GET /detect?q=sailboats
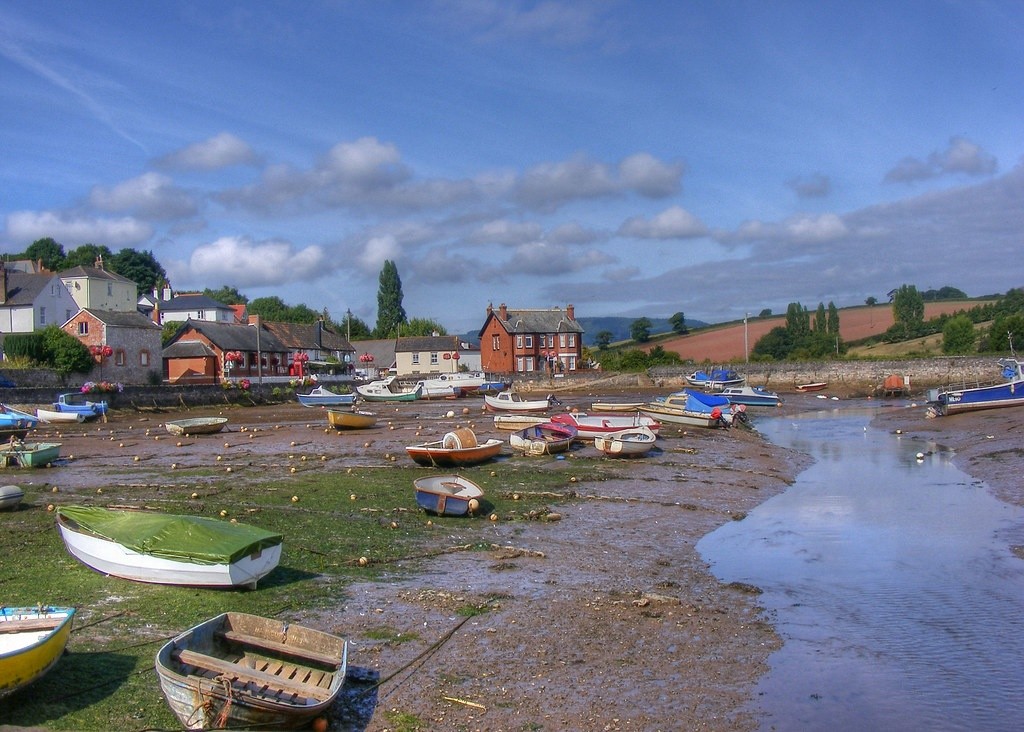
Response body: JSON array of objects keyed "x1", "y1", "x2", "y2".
[{"x1": 713, "y1": 310, "x2": 781, "y2": 405}]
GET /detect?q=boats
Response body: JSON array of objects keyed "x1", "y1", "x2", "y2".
[
  {"x1": 0, "y1": 484, "x2": 24, "y2": 510},
  {"x1": 482, "y1": 392, "x2": 561, "y2": 413},
  {"x1": 0, "y1": 404, "x2": 39, "y2": 441},
  {"x1": 406, "y1": 426, "x2": 504, "y2": 467},
  {"x1": 53, "y1": 392, "x2": 108, "y2": 418},
  {"x1": 592, "y1": 402, "x2": 647, "y2": 411},
  {"x1": 414, "y1": 473, "x2": 485, "y2": 517},
  {"x1": 0, "y1": 442, "x2": 62, "y2": 468},
  {"x1": 356, "y1": 371, "x2": 511, "y2": 401},
  {"x1": 935, "y1": 358, "x2": 1024, "y2": 416},
  {"x1": 154, "y1": 611, "x2": 347, "y2": 732},
  {"x1": 795, "y1": 381, "x2": 827, "y2": 393},
  {"x1": 295, "y1": 386, "x2": 356, "y2": 407},
  {"x1": 327, "y1": 406, "x2": 376, "y2": 429},
  {"x1": 0, "y1": 607, "x2": 75, "y2": 698},
  {"x1": 884, "y1": 374, "x2": 905, "y2": 389},
  {"x1": 636, "y1": 368, "x2": 747, "y2": 427},
  {"x1": 494, "y1": 411, "x2": 662, "y2": 455},
  {"x1": 35, "y1": 407, "x2": 85, "y2": 422},
  {"x1": 55, "y1": 505, "x2": 283, "y2": 589},
  {"x1": 165, "y1": 416, "x2": 228, "y2": 435}
]
